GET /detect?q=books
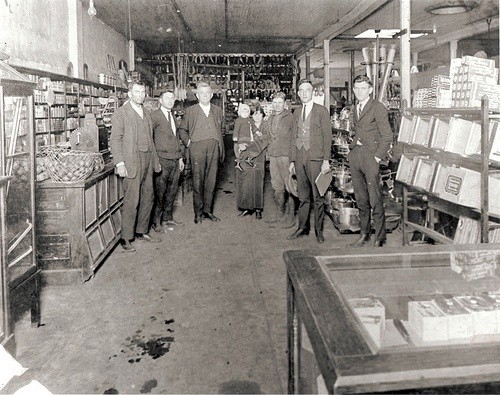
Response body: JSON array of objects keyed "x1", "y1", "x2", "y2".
[{"x1": 315, "y1": 166, "x2": 332, "y2": 196}]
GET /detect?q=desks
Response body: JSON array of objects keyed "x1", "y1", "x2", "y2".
[{"x1": 282, "y1": 243, "x2": 500, "y2": 395}]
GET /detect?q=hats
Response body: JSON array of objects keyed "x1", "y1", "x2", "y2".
[
  {"x1": 285, "y1": 169, "x2": 298, "y2": 198},
  {"x1": 237, "y1": 104, "x2": 251, "y2": 116}
]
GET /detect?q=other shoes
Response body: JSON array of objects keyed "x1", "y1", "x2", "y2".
[
  {"x1": 357, "y1": 232, "x2": 370, "y2": 243},
  {"x1": 234, "y1": 162, "x2": 244, "y2": 171},
  {"x1": 287, "y1": 226, "x2": 310, "y2": 239},
  {"x1": 194, "y1": 214, "x2": 202, "y2": 224},
  {"x1": 256, "y1": 209, "x2": 262, "y2": 219},
  {"x1": 374, "y1": 240, "x2": 384, "y2": 247},
  {"x1": 245, "y1": 157, "x2": 254, "y2": 167},
  {"x1": 317, "y1": 235, "x2": 324, "y2": 242},
  {"x1": 120, "y1": 238, "x2": 136, "y2": 252},
  {"x1": 152, "y1": 221, "x2": 164, "y2": 233},
  {"x1": 137, "y1": 232, "x2": 161, "y2": 243},
  {"x1": 162, "y1": 219, "x2": 185, "y2": 226},
  {"x1": 204, "y1": 212, "x2": 221, "y2": 222},
  {"x1": 239, "y1": 209, "x2": 254, "y2": 217}
]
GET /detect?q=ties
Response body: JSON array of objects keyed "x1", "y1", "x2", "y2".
[
  {"x1": 167, "y1": 110, "x2": 171, "y2": 127},
  {"x1": 358, "y1": 104, "x2": 363, "y2": 118},
  {"x1": 303, "y1": 104, "x2": 306, "y2": 121}
]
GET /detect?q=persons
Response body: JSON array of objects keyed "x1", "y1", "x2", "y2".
[
  {"x1": 233, "y1": 104, "x2": 262, "y2": 171},
  {"x1": 110, "y1": 81, "x2": 163, "y2": 251},
  {"x1": 288, "y1": 80, "x2": 333, "y2": 243},
  {"x1": 266, "y1": 91, "x2": 299, "y2": 228},
  {"x1": 347, "y1": 75, "x2": 393, "y2": 247},
  {"x1": 148, "y1": 90, "x2": 184, "y2": 233},
  {"x1": 178, "y1": 82, "x2": 227, "y2": 225},
  {"x1": 234, "y1": 104, "x2": 269, "y2": 219}
]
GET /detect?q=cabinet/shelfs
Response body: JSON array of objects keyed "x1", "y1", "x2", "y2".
[
  {"x1": 394, "y1": 96, "x2": 500, "y2": 249},
  {"x1": 0, "y1": 56, "x2": 132, "y2": 340}
]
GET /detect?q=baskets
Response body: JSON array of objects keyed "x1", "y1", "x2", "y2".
[{"x1": 43, "y1": 148, "x2": 106, "y2": 182}]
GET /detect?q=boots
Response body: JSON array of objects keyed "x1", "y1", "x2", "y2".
[
  {"x1": 282, "y1": 198, "x2": 296, "y2": 228},
  {"x1": 266, "y1": 190, "x2": 285, "y2": 222}
]
